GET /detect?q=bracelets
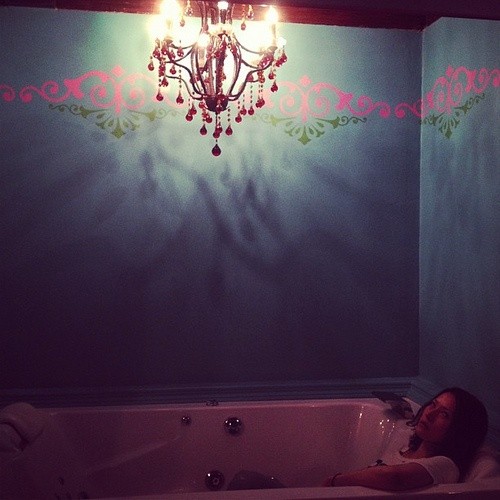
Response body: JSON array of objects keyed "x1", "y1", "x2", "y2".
[{"x1": 331, "y1": 472, "x2": 343, "y2": 486}]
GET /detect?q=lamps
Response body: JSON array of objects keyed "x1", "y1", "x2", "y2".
[{"x1": 147, "y1": 0, "x2": 287, "y2": 156}]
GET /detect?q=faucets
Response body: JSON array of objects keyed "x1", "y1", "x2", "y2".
[{"x1": 373, "y1": 389, "x2": 413, "y2": 422}]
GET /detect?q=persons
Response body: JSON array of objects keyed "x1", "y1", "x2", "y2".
[{"x1": 222, "y1": 386, "x2": 488, "y2": 491}]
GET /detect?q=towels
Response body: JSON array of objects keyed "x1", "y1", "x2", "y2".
[{"x1": 0, "y1": 402, "x2": 48, "y2": 452}]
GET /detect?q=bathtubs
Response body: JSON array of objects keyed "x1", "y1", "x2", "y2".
[{"x1": 0, "y1": 397, "x2": 500, "y2": 500}]
